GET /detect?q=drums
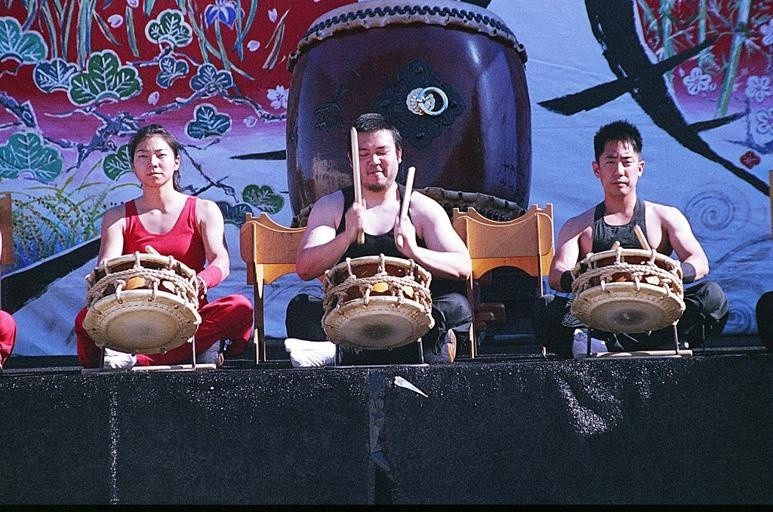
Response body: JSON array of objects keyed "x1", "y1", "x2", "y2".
[
  {"x1": 284, "y1": 0, "x2": 531, "y2": 227},
  {"x1": 320, "y1": 253, "x2": 435, "y2": 355},
  {"x1": 80, "y1": 250, "x2": 201, "y2": 357},
  {"x1": 570, "y1": 247, "x2": 686, "y2": 336}
]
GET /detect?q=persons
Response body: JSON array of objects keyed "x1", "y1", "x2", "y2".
[
  {"x1": 68, "y1": 122, "x2": 254, "y2": 371},
  {"x1": 530, "y1": 121, "x2": 730, "y2": 357},
  {"x1": 280, "y1": 110, "x2": 476, "y2": 366}
]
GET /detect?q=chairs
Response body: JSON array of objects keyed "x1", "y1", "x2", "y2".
[
  {"x1": 238, "y1": 212, "x2": 325, "y2": 366},
  {"x1": 451, "y1": 201, "x2": 557, "y2": 360}
]
